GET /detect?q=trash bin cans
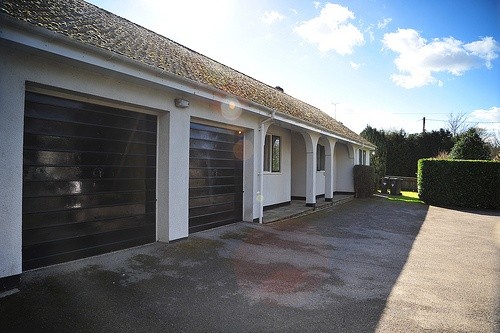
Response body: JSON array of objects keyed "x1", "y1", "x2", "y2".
[
  {"x1": 389, "y1": 178, "x2": 404, "y2": 196},
  {"x1": 381, "y1": 177, "x2": 390, "y2": 194}
]
